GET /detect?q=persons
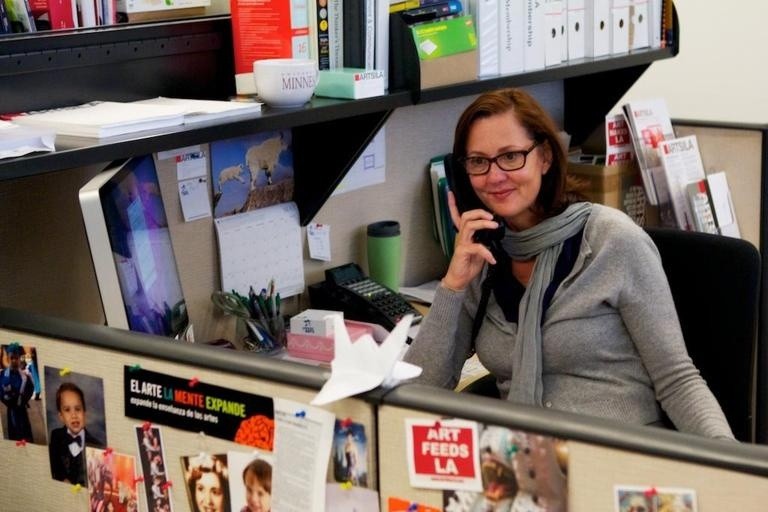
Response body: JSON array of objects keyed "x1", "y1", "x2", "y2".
[
  {"x1": 0, "y1": 345, "x2": 35, "y2": 444},
  {"x1": 344, "y1": 431, "x2": 362, "y2": 487},
  {"x1": 184, "y1": 452, "x2": 231, "y2": 511},
  {"x1": 49, "y1": 382, "x2": 102, "y2": 487},
  {"x1": 239, "y1": 458, "x2": 272, "y2": 512},
  {"x1": 399, "y1": 86, "x2": 738, "y2": 442},
  {"x1": 140, "y1": 429, "x2": 170, "y2": 511},
  {"x1": 89, "y1": 476, "x2": 136, "y2": 512}
]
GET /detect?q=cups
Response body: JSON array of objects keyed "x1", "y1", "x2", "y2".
[{"x1": 251, "y1": 57, "x2": 323, "y2": 110}]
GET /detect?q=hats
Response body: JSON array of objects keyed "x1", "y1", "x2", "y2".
[{"x1": 4, "y1": 346, "x2": 26, "y2": 356}]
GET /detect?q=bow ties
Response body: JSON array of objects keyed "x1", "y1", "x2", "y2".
[{"x1": 66, "y1": 432, "x2": 83, "y2": 448}]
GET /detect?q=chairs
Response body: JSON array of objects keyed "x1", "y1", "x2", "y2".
[{"x1": 459, "y1": 228, "x2": 760, "y2": 443}]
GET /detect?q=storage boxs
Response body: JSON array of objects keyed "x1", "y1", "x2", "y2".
[{"x1": 566, "y1": 160, "x2": 659, "y2": 228}]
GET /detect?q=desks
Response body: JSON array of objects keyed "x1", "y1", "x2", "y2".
[{"x1": 0, "y1": 272, "x2": 768, "y2": 512}]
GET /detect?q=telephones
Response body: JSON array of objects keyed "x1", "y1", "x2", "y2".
[{"x1": 443, "y1": 152, "x2": 506, "y2": 242}]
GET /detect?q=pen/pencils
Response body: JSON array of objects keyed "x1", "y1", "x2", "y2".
[{"x1": 232, "y1": 279, "x2": 281, "y2": 349}]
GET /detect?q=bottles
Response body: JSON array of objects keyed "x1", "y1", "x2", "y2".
[{"x1": 366, "y1": 218, "x2": 401, "y2": 299}]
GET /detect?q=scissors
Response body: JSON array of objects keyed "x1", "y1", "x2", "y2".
[{"x1": 211, "y1": 292, "x2": 251, "y2": 319}]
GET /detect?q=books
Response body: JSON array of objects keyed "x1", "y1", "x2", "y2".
[
  {"x1": 0, "y1": 1, "x2": 211, "y2": 36},
  {"x1": 618, "y1": 96, "x2": 742, "y2": 239},
  {"x1": 227, "y1": 1, "x2": 392, "y2": 97},
  {"x1": 0, "y1": 95, "x2": 266, "y2": 153}
]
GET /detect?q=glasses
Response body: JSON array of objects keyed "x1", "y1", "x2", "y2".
[{"x1": 457, "y1": 136, "x2": 544, "y2": 177}]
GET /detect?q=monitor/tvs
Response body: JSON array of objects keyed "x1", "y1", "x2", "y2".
[{"x1": 76, "y1": 152, "x2": 192, "y2": 341}]
